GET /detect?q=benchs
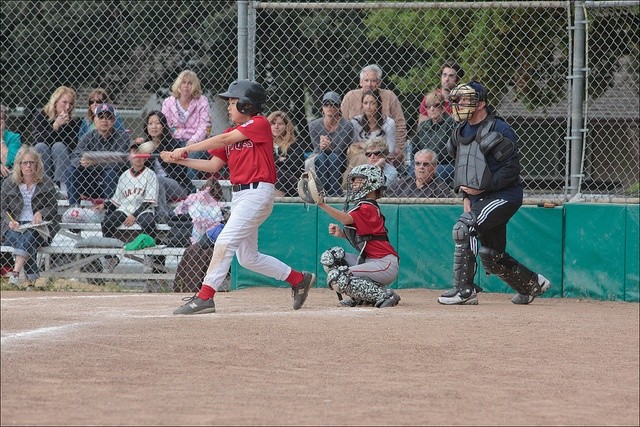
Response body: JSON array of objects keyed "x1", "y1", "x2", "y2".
[
  {"x1": 1, "y1": 245, "x2": 186, "y2": 280},
  {"x1": 58, "y1": 222, "x2": 171, "y2": 232},
  {"x1": 56, "y1": 200, "x2": 93, "y2": 217},
  {"x1": 191, "y1": 178, "x2": 234, "y2": 204}
]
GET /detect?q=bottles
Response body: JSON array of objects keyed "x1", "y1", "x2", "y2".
[{"x1": 404, "y1": 139, "x2": 413, "y2": 160}]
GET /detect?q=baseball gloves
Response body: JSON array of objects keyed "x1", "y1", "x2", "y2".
[{"x1": 297, "y1": 170, "x2": 325, "y2": 204}]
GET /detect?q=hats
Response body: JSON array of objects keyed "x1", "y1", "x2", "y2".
[
  {"x1": 322, "y1": 91, "x2": 342, "y2": 104},
  {"x1": 126, "y1": 138, "x2": 145, "y2": 152},
  {"x1": 96, "y1": 103, "x2": 114, "y2": 117}
]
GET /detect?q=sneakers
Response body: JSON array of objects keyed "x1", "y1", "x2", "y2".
[
  {"x1": 291, "y1": 271, "x2": 315, "y2": 310},
  {"x1": 173, "y1": 291, "x2": 215, "y2": 314},
  {"x1": 337, "y1": 297, "x2": 357, "y2": 306},
  {"x1": 143, "y1": 257, "x2": 155, "y2": 273},
  {"x1": 7, "y1": 275, "x2": 20, "y2": 289},
  {"x1": 103, "y1": 256, "x2": 120, "y2": 273},
  {"x1": 437, "y1": 287, "x2": 479, "y2": 305},
  {"x1": 511, "y1": 273, "x2": 552, "y2": 304},
  {"x1": 17, "y1": 274, "x2": 29, "y2": 289},
  {"x1": 379, "y1": 289, "x2": 401, "y2": 308}
]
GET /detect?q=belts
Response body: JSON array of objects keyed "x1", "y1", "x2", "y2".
[{"x1": 230, "y1": 182, "x2": 259, "y2": 191}]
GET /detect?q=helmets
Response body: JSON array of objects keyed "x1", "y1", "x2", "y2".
[
  {"x1": 345, "y1": 164, "x2": 386, "y2": 202},
  {"x1": 218, "y1": 79, "x2": 267, "y2": 114},
  {"x1": 448, "y1": 81, "x2": 488, "y2": 124}
]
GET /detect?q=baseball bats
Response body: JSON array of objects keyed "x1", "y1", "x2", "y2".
[{"x1": 82, "y1": 150, "x2": 189, "y2": 162}]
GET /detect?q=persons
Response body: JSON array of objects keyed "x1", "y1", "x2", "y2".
[
  {"x1": 160, "y1": 80, "x2": 316, "y2": 315},
  {"x1": 342, "y1": 65, "x2": 408, "y2": 167},
  {"x1": 341, "y1": 89, "x2": 399, "y2": 192},
  {"x1": 383, "y1": 150, "x2": 459, "y2": 199},
  {"x1": 65, "y1": 104, "x2": 130, "y2": 232},
  {"x1": 268, "y1": 111, "x2": 305, "y2": 196},
  {"x1": 32, "y1": 85, "x2": 82, "y2": 198},
  {"x1": 363, "y1": 139, "x2": 400, "y2": 198},
  {"x1": 298, "y1": 164, "x2": 401, "y2": 308},
  {"x1": 406, "y1": 92, "x2": 461, "y2": 187},
  {"x1": 1, "y1": 146, "x2": 58, "y2": 290},
  {"x1": 308, "y1": 92, "x2": 354, "y2": 197},
  {"x1": 140, "y1": 110, "x2": 196, "y2": 248},
  {"x1": 437, "y1": 80, "x2": 550, "y2": 304},
  {"x1": 77, "y1": 88, "x2": 125, "y2": 145},
  {"x1": 102, "y1": 140, "x2": 166, "y2": 243},
  {"x1": 418, "y1": 62, "x2": 465, "y2": 127},
  {"x1": 161, "y1": 70, "x2": 212, "y2": 179},
  {"x1": 0, "y1": 111, "x2": 21, "y2": 276}
]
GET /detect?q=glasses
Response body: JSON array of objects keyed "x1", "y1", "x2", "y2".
[
  {"x1": 21, "y1": 161, "x2": 36, "y2": 165},
  {"x1": 425, "y1": 103, "x2": 441, "y2": 108},
  {"x1": 365, "y1": 151, "x2": 385, "y2": 157},
  {"x1": 415, "y1": 162, "x2": 433, "y2": 167},
  {"x1": 90, "y1": 99, "x2": 102, "y2": 104},
  {"x1": 96, "y1": 113, "x2": 112, "y2": 119},
  {"x1": 323, "y1": 101, "x2": 338, "y2": 107}
]
